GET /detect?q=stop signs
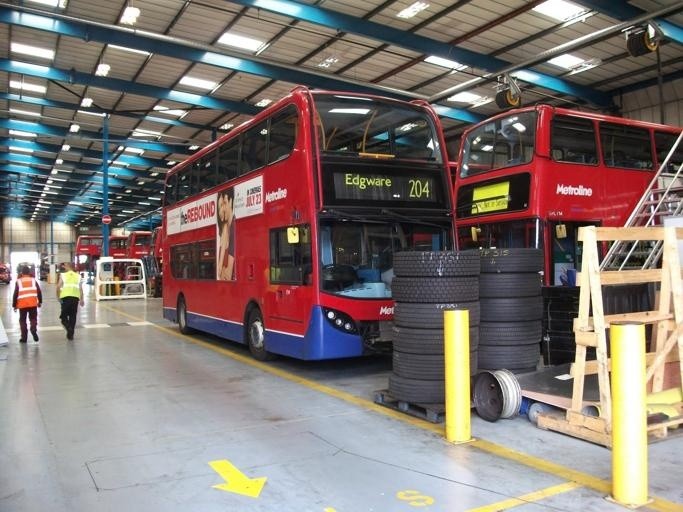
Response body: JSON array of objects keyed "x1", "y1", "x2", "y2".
[{"x1": 102, "y1": 216, "x2": 112, "y2": 224}]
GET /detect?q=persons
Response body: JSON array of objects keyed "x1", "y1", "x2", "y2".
[
  {"x1": 55, "y1": 262, "x2": 85, "y2": 341},
  {"x1": 217, "y1": 191, "x2": 230, "y2": 281},
  {"x1": 223, "y1": 187, "x2": 236, "y2": 281},
  {"x1": 12, "y1": 267, "x2": 43, "y2": 344}
]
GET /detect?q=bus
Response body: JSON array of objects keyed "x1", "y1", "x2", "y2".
[
  {"x1": 72, "y1": 225, "x2": 164, "y2": 296},
  {"x1": 453, "y1": 105, "x2": 683, "y2": 287},
  {"x1": 158, "y1": 90, "x2": 454, "y2": 360}
]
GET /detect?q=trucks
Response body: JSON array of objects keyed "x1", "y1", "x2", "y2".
[
  {"x1": 17, "y1": 261, "x2": 71, "y2": 281},
  {"x1": 0, "y1": 262, "x2": 11, "y2": 284}
]
